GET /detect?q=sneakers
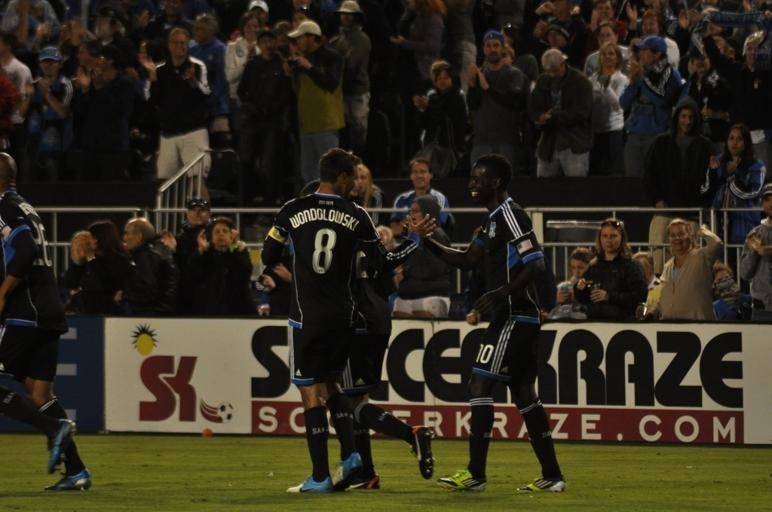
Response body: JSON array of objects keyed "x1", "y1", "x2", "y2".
[
  {"x1": 286, "y1": 474, "x2": 334, "y2": 494},
  {"x1": 515, "y1": 475, "x2": 567, "y2": 493},
  {"x1": 48, "y1": 418, "x2": 76, "y2": 474},
  {"x1": 436, "y1": 469, "x2": 487, "y2": 492},
  {"x1": 332, "y1": 451, "x2": 379, "y2": 492},
  {"x1": 407, "y1": 425, "x2": 434, "y2": 479},
  {"x1": 44, "y1": 467, "x2": 92, "y2": 491}
]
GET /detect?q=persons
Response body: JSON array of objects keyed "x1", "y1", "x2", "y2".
[
  {"x1": 57, "y1": 230, "x2": 97, "y2": 311},
  {"x1": 389, "y1": 157, "x2": 451, "y2": 245},
  {"x1": 672, "y1": 0, "x2": 772, "y2": 141},
  {"x1": 401, "y1": 154, "x2": 568, "y2": 492},
  {"x1": 300, "y1": 179, "x2": 434, "y2": 489},
  {"x1": 189, "y1": 216, "x2": 255, "y2": 315},
  {"x1": 632, "y1": 251, "x2": 662, "y2": 300},
  {"x1": 348, "y1": 164, "x2": 391, "y2": 228},
  {"x1": 547, "y1": 247, "x2": 595, "y2": 321},
  {"x1": 381, "y1": 194, "x2": 451, "y2": 318},
  {"x1": 172, "y1": 198, "x2": 216, "y2": 318},
  {"x1": 572, "y1": 218, "x2": 648, "y2": 323},
  {"x1": 1, "y1": 1, "x2": 371, "y2": 207},
  {"x1": 644, "y1": 95, "x2": 717, "y2": 279},
  {"x1": 369, "y1": 1, "x2": 672, "y2": 175},
  {"x1": 89, "y1": 218, "x2": 127, "y2": 317},
  {"x1": 113, "y1": 216, "x2": 178, "y2": 317},
  {"x1": 0, "y1": 152, "x2": 92, "y2": 492},
  {"x1": 657, "y1": 216, "x2": 724, "y2": 322},
  {"x1": 739, "y1": 182, "x2": 772, "y2": 325},
  {"x1": 712, "y1": 260, "x2": 752, "y2": 323},
  {"x1": 698, "y1": 123, "x2": 766, "y2": 292},
  {"x1": 260, "y1": 148, "x2": 436, "y2": 493}
]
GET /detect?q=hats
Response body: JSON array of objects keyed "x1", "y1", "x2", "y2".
[
  {"x1": 757, "y1": 184, "x2": 772, "y2": 199},
  {"x1": 635, "y1": 36, "x2": 667, "y2": 52},
  {"x1": 540, "y1": 49, "x2": 569, "y2": 71},
  {"x1": 249, "y1": 1, "x2": 270, "y2": 14},
  {"x1": 287, "y1": 21, "x2": 322, "y2": 39},
  {"x1": 185, "y1": 199, "x2": 211, "y2": 211},
  {"x1": 483, "y1": 31, "x2": 505, "y2": 45},
  {"x1": 335, "y1": 1, "x2": 363, "y2": 14},
  {"x1": 38, "y1": 47, "x2": 64, "y2": 63}
]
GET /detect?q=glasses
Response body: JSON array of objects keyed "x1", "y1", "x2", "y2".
[
  {"x1": 189, "y1": 201, "x2": 205, "y2": 206},
  {"x1": 601, "y1": 221, "x2": 622, "y2": 228}
]
